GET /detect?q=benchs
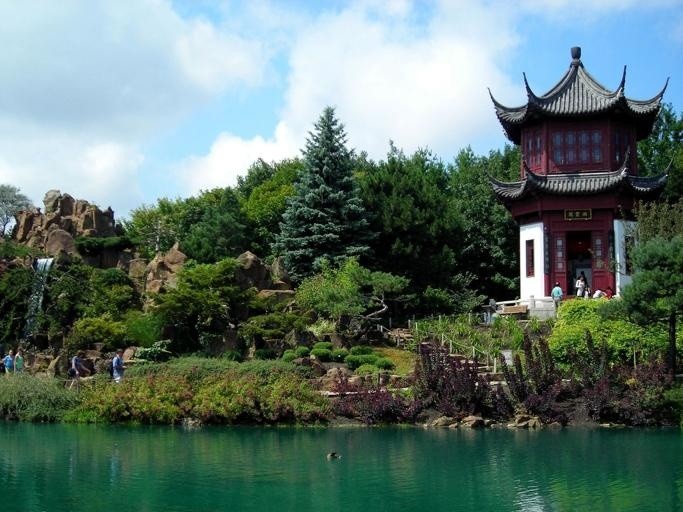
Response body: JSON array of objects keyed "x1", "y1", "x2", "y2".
[{"x1": 499, "y1": 305, "x2": 528, "y2": 320}]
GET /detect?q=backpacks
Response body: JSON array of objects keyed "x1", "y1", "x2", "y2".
[{"x1": 110, "y1": 357, "x2": 119, "y2": 375}]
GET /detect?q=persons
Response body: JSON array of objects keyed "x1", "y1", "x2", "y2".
[
  {"x1": 550, "y1": 282, "x2": 563, "y2": 312},
  {"x1": 112, "y1": 348, "x2": 128, "y2": 384},
  {"x1": 0, "y1": 350, "x2": 15, "y2": 375},
  {"x1": 576, "y1": 272, "x2": 614, "y2": 300},
  {"x1": 68, "y1": 351, "x2": 91, "y2": 395},
  {"x1": 15, "y1": 348, "x2": 25, "y2": 373}
]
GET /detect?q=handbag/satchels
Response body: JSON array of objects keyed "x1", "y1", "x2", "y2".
[{"x1": 67, "y1": 368, "x2": 76, "y2": 377}]
